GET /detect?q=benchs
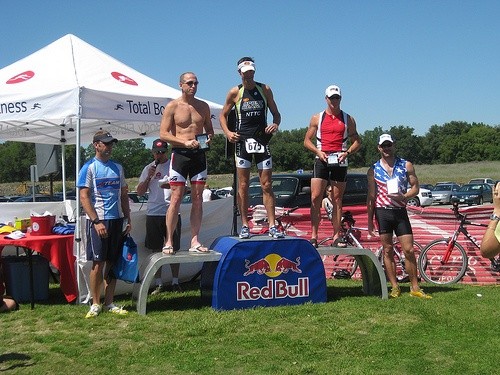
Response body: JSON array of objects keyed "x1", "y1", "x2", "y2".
[{"x1": 129, "y1": 234, "x2": 389, "y2": 314}]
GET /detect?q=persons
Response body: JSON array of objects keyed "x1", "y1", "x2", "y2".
[
  {"x1": 302, "y1": 85, "x2": 364, "y2": 248},
  {"x1": 158, "y1": 71, "x2": 212, "y2": 256},
  {"x1": 479, "y1": 180, "x2": 500, "y2": 259},
  {"x1": 365, "y1": 133, "x2": 435, "y2": 302},
  {"x1": 137, "y1": 137, "x2": 184, "y2": 297},
  {"x1": 74, "y1": 130, "x2": 133, "y2": 319},
  {"x1": 321, "y1": 184, "x2": 334, "y2": 210},
  {"x1": 220, "y1": 56, "x2": 286, "y2": 240}
]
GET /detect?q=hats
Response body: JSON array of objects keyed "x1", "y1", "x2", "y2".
[
  {"x1": 376, "y1": 134, "x2": 394, "y2": 147},
  {"x1": 151, "y1": 139, "x2": 168, "y2": 153},
  {"x1": 237, "y1": 61, "x2": 256, "y2": 73},
  {"x1": 92, "y1": 132, "x2": 118, "y2": 144},
  {"x1": 324, "y1": 85, "x2": 341, "y2": 98}
]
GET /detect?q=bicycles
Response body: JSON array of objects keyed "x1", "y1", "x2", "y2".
[
  {"x1": 313, "y1": 211, "x2": 426, "y2": 282},
  {"x1": 417, "y1": 198, "x2": 500, "y2": 287}
]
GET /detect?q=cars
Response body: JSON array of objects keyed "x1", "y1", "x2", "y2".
[{"x1": 232, "y1": 167, "x2": 498, "y2": 216}]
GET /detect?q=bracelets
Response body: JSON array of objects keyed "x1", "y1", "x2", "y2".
[
  {"x1": 346, "y1": 150, "x2": 351, "y2": 155},
  {"x1": 489, "y1": 212, "x2": 500, "y2": 221},
  {"x1": 92, "y1": 218, "x2": 100, "y2": 224}
]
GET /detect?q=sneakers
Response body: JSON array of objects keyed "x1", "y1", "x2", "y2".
[
  {"x1": 268, "y1": 226, "x2": 285, "y2": 239},
  {"x1": 102, "y1": 302, "x2": 129, "y2": 316},
  {"x1": 409, "y1": 286, "x2": 433, "y2": 301},
  {"x1": 85, "y1": 304, "x2": 101, "y2": 318},
  {"x1": 389, "y1": 286, "x2": 401, "y2": 298},
  {"x1": 239, "y1": 225, "x2": 251, "y2": 239}
]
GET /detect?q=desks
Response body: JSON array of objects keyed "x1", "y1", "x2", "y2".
[{"x1": 0, "y1": 224, "x2": 75, "y2": 309}]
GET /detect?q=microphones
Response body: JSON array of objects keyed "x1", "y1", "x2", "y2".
[{"x1": 149, "y1": 160, "x2": 159, "y2": 179}]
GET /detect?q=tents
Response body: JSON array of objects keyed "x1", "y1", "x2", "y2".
[{"x1": 0, "y1": 32, "x2": 233, "y2": 302}]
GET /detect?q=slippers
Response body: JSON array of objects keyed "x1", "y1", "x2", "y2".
[
  {"x1": 309, "y1": 239, "x2": 318, "y2": 248},
  {"x1": 162, "y1": 246, "x2": 176, "y2": 255},
  {"x1": 188, "y1": 244, "x2": 210, "y2": 254},
  {"x1": 331, "y1": 238, "x2": 347, "y2": 248}
]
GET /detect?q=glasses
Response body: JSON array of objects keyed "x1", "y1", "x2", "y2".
[
  {"x1": 182, "y1": 81, "x2": 199, "y2": 86},
  {"x1": 326, "y1": 96, "x2": 340, "y2": 99},
  {"x1": 379, "y1": 143, "x2": 393, "y2": 147},
  {"x1": 326, "y1": 189, "x2": 332, "y2": 192}
]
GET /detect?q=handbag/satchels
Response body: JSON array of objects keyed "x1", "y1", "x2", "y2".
[{"x1": 107, "y1": 231, "x2": 139, "y2": 284}]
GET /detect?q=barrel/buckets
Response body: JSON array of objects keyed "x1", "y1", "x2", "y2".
[{"x1": 29, "y1": 214, "x2": 57, "y2": 236}]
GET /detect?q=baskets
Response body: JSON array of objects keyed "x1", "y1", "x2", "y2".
[{"x1": 1, "y1": 254, "x2": 50, "y2": 304}]
